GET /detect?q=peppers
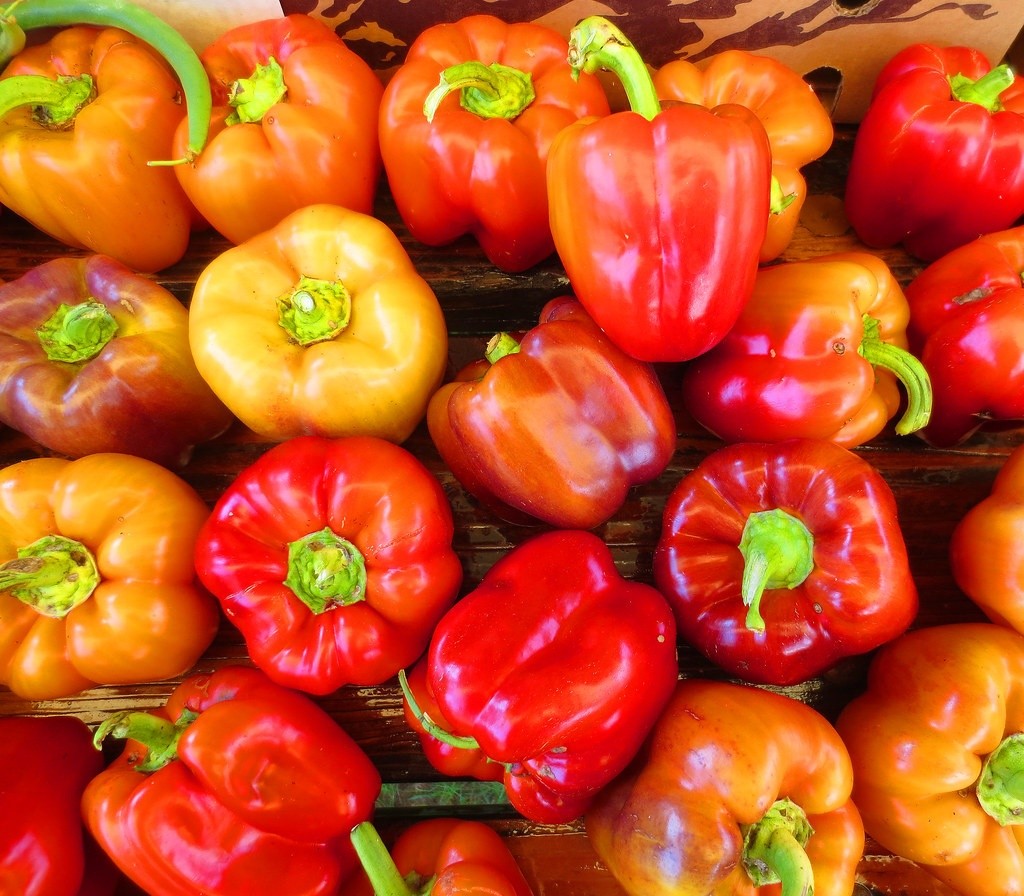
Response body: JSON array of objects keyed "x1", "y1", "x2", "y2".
[{"x1": 0, "y1": 0, "x2": 1024, "y2": 896}]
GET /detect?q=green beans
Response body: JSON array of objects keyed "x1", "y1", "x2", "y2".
[{"x1": 7, "y1": 0, "x2": 212, "y2": 166}]
